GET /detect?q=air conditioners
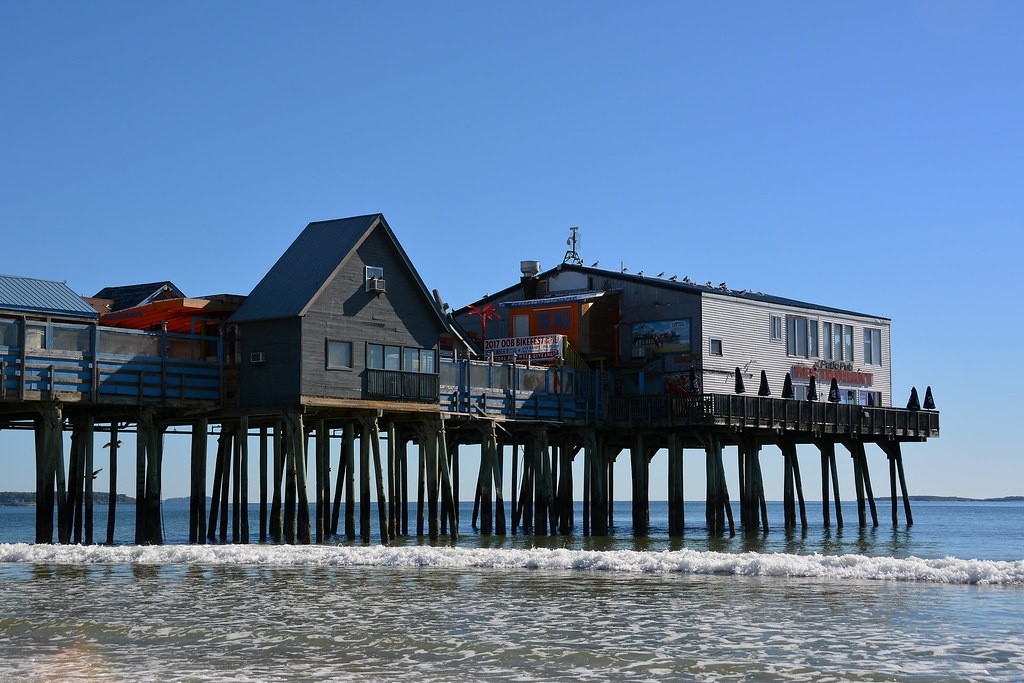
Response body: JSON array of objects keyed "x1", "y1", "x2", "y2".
[
  {"x1": 251, "y1": 352, "x2": 265, "y2": 362},
  {"x1": 370, "y1": 279, "x2": 386, "y2": 291}
]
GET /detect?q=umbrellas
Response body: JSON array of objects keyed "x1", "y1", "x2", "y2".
[
  {"x1": 924, "y1": 386, "x2": 936, "y2": 409},
  {"x1": 690, "y1": 366, "x2": 699, "y2": 392},
  {"x1": 758, "y1": 369, "x2": 771, "y2": 396},
  {"x1": 807, "y1": 375, "x2": 818, "y2": 399},
  {"x1": 828, "y1": 377, "x2": 841, "y2": 402},
  {"x1": 782, "y1": 372, "x2": 794, "y2": 398},
  {"x1": 735, "y1": 367, "x2": 745, "y2": 393},
  {"x1": 907, "y1": 386, "x2": 920, "y2": 411}
]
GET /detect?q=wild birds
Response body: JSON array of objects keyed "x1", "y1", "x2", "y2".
[
  {"x1": 683, "y1": 276, "x2": 766, "y2": 297},
  {"x1": 591, "y1": 260, "x2": 599, "y2": 268},
  {"x1": 656, "y1": 272, "x2": 664, "y2": 278},
  {"x1": 668, "y1": 275, "x2": 678, "y2": 281},
  {"x1": 637, "y1": 271, "x2": 644, "y2": 276},
  {"x1": 621, "y1": 268, "x2": 630, "y2": 274}
]
